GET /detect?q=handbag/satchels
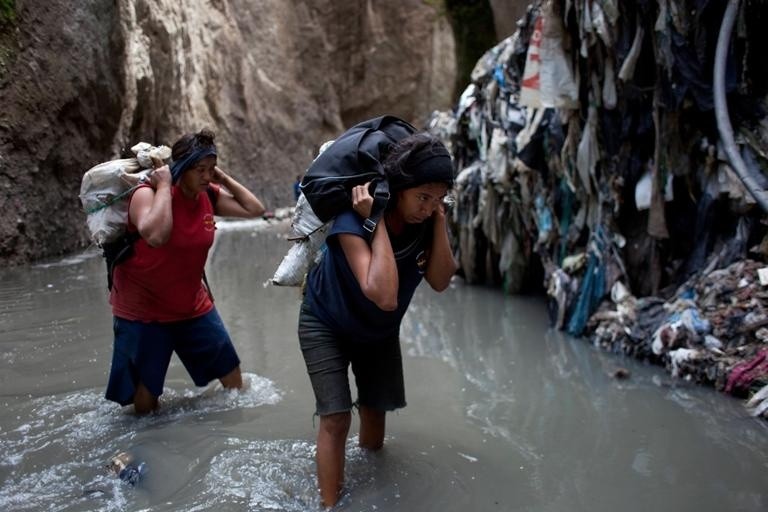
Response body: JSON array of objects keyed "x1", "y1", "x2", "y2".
[{"x1": 302, "y1": 114, "x2": 417, "y2": 223}]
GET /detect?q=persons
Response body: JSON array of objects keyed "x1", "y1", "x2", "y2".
[
  {"x1": 297, "y1": 130, "x2": 456, "y2": 509},
  {"x1": 104, "y1": 126, "x2": 265, "y2": 418}
]
